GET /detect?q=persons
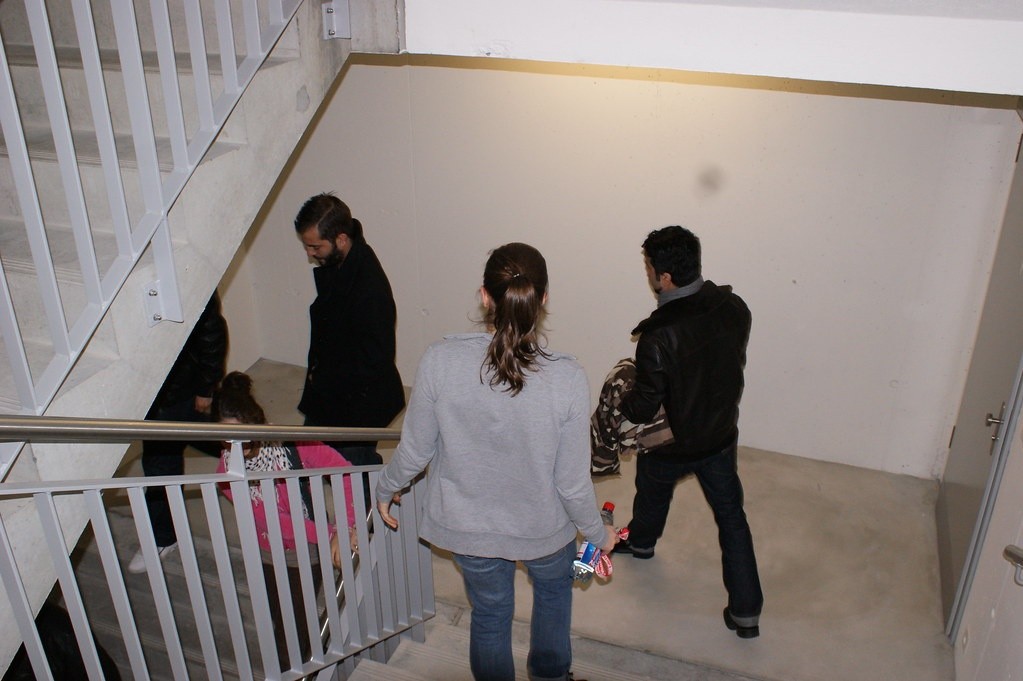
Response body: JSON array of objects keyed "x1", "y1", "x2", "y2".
[
  {"x1": 126, "y1": 291, "x2": 224, "y2": 575},
  {"x1": 294, "y1": 193, "x2": 406, "y2": 536},
  {"x1": 375, "y1": 242, "x2": 620, "y2": 681},
  {"x1": 210, "y1": 371, "x2": 356, "y2": 674},
  {"x1": 610, "y1": 226, "x2": 764, "y2": 640}
]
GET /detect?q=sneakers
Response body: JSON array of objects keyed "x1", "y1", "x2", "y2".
[{"x1": 128, "y1": 540, "x2": 178, "y2": 574}]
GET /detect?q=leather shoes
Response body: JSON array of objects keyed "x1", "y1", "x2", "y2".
[
  {"x1": 723, "y1": 606, "x2": 759, "y2": 638},
  {"x1": 612, "y1": 539, "x2": 654, "y2": 558}
]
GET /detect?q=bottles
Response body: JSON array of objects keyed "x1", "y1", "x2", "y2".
[{"x1": 568, "y1": 502, "x2": 615, "y2": 583}]
